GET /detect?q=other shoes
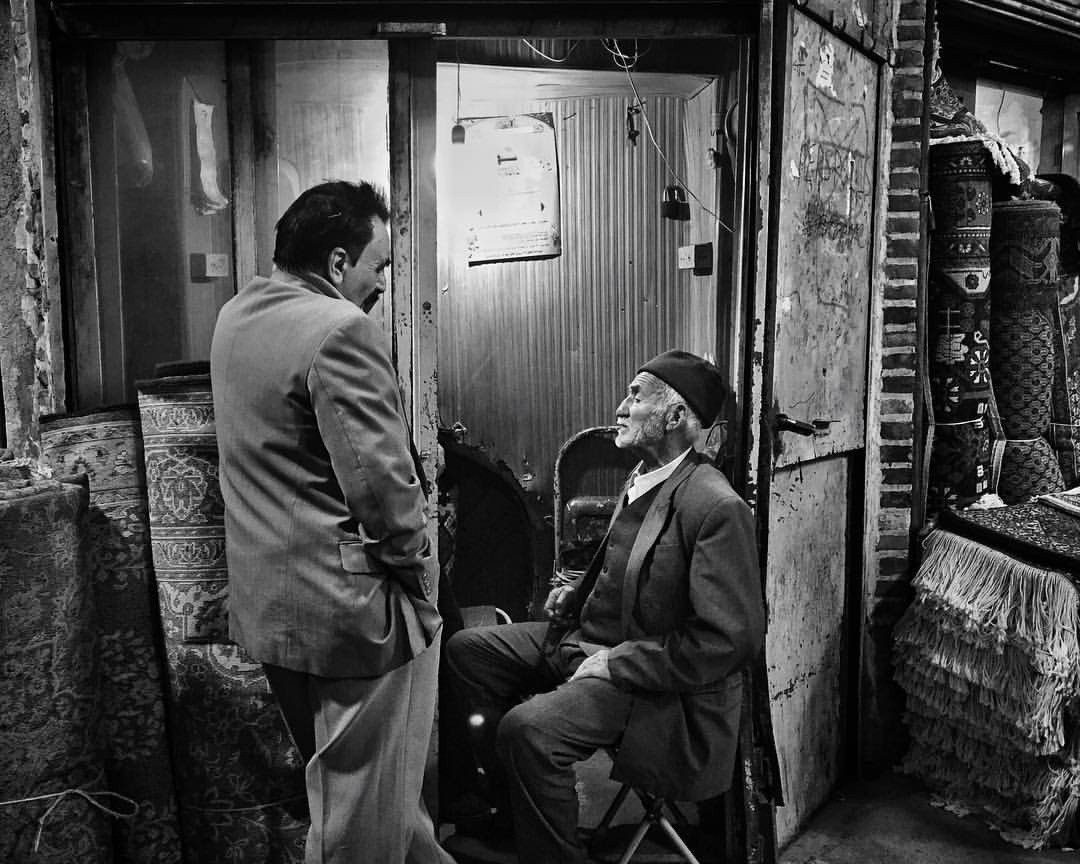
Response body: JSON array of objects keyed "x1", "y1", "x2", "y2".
[{"x1": 455, "y1": 809, "x2": 515, "y2": 842}]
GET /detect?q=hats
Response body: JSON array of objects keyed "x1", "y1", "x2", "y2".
[{"x1": 637, "y1": 350, "x2": 726, "y2": 429}]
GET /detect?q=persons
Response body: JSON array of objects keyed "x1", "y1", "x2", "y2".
[
  {"x1": 210, "y1": 177, "x2": 445, "y2": 864},
  {"x1": 439, "y1": 348, "x2": 765, "y2": 864}
]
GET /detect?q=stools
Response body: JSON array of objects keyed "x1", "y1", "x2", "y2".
[{"x1": 590, "y1": 743, "x2": 701, "y2": 864}]
